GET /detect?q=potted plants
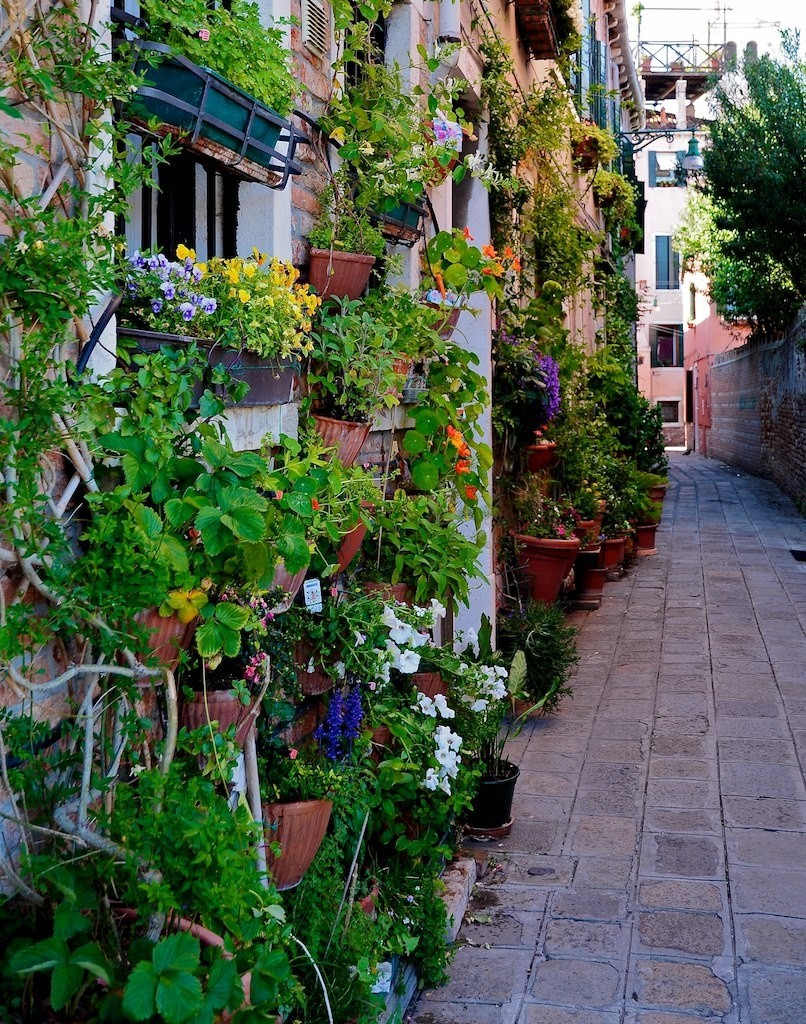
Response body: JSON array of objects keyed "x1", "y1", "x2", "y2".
[
  {"x1": 641, "y1": 55, "x2": 653, "y2": 71},
  {"x1": 671, "y1": 61, "x2": 686, "y2": 71},
  {"x1": 711, "y1": 54, "x2": 722, "y2": 70},
  {"x1": 577, "y1": 125, "x2": 645, "y2": 252},
  {"x1": 625, "y1": 472, "x2": 669, "y2": 551}
]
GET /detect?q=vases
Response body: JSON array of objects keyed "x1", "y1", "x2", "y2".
[{"x1": 1, "y1": 13, "x2": 637, "y2": 1023}]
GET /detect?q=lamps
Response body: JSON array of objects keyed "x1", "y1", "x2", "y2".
[
  {"x1": 621, "y1": 130, "x2": 705, "y2": 170},
  {"x1": 638, "y1": 294, "x2": 661, "y2": 313}
]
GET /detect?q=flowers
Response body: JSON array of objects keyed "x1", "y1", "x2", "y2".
[{"x1": 0, "y1": 0, "x2": 620, "y2": 1024}]
